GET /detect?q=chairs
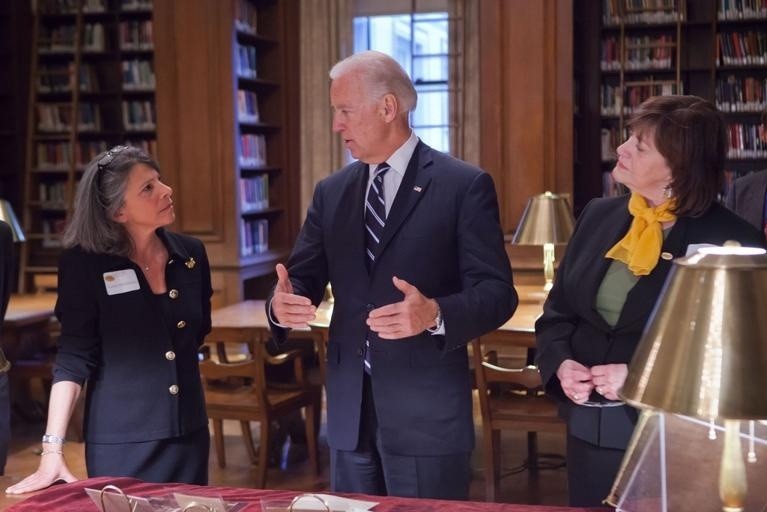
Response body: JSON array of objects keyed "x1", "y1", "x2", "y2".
[
  {"x1": 190, "y1": 325, "x2": 323, "y2": 487},
  {"x1": 472, "y1": 329, "x2": 572, "y2": 501}
]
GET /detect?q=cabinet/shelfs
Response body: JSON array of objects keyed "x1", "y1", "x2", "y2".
[
  {"x1": 572, "y1": 0, "x2": 766, "y2": 232},
  {"x1": 152, "y1": 1, "x2": 303, "y2": 269},
  {"x1": 0, "y1": 2, "x2": 157, "y2": 288}
]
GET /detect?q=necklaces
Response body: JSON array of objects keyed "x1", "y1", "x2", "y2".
[{"x1": 128, "y1": 238, "x2": 163, "y2": 271}]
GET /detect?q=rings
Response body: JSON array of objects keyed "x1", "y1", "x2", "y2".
[{"x1": 571, "y1": 391, "x2": 582, "y2": 401}]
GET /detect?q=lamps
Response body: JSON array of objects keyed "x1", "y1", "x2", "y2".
[
  {"x1": 614, "y1": 244, "x2": 767, "y2": 512},
  {"x1": 512, "y1": 189, "x2": 578, "y2": 299}
]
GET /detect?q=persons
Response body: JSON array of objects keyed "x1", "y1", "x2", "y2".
[
  {"x1": 264, "y1": 49, "x2": 521, "y2": 501},
  {"x1": 534, "y1": 96, "x2": 766, "y2": 508},
  {"x1": 3, "y1": 142, "x2": 213, "y2": 499},
  {"x1": 726, "y1": 167, "x2": 766, "y2": 237}
]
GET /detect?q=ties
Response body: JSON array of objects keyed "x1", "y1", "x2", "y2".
[{"x1": 365, "y1": 162, "x2": 391, "y2": 374}]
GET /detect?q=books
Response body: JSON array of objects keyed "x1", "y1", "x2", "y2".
[
  {"x1": 235, "y1": 3, "x2": 268, "y2": 255},
  {"x1": 31, "y1": 0, "x2": 158, "y2": 205},
  {"x1": 597, "y1": 0, "x2": 766, "y2": 202}
]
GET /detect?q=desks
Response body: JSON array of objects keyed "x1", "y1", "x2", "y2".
[
  {"x1": 0, "y1": 477, "x2": 622, "y2": 512},
  {"x1": 194, "y1": 287, "x2": 569, "y2": 352},
  {"x1": 0, "y1": 291, "x2": 101, "y2": 444}
]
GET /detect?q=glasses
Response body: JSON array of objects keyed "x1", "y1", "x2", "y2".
[{"x1": 97, "y1": 145, "x2": 129, "y2": 183}]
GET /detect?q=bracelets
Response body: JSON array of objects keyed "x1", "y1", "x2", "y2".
[
  {"x1": 40, "y1": 434, "x2": 65, "y2": 445},
  {"x1": 40, "y1": 451, "x2": 64, "y2": 456}
]
